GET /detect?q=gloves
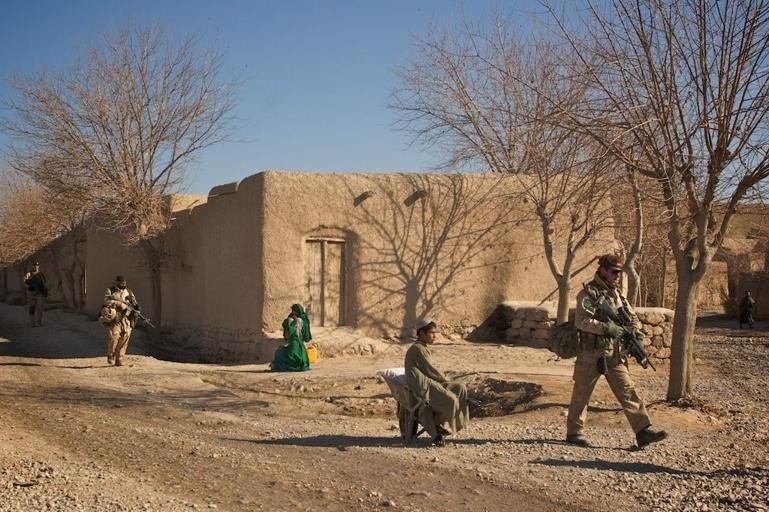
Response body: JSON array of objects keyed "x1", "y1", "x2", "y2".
[{"x1": 606, "y1": 321, "x2": 624, "y2": 338}]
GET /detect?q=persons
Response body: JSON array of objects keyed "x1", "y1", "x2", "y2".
[
  {"x1": 23, "y1": 260, "x2": 48, "y2": 327},
  {"x1": 566, "y1": 253, "x2": 667, "y2": 450},
  {"x1": 738, "y1": 287, "x2": 756, "y2": 329},
  {"x1": 273, "y1": 302, "x2": 313, "y2": 372},
  {"x1": 103, "y1": 274, "x2": 142, "y2": 366},
  {"x1": 403, "y1": 317, "x2": 468, "y2": 440}
]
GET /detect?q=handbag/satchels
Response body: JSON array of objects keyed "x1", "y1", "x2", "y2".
[
  {"x1": 546, "y1": 325, "x2": 577, "y2": 359},
  {"x1": 99, "y1": 307, "x2": 116, "y2": 324}
]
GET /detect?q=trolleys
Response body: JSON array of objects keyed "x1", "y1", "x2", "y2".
[{"x1": 376, "y1": 365, "x2": 483, "y2": 446}]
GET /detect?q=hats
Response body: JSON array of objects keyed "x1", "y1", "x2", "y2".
[
  {"x1": 599, "y1": 255, "x2": 624, "y2": 270},
  {"x1": 117, "y1": 275, "x2": 127, "y2": 281},
  {"x1": 417, "y1": 318, "x2": 431, "y2": 329}
]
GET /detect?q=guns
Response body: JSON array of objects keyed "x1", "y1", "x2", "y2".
[
  {"x1": 123, "y1": 301, "x2": 156, "y2": 331},
  {"x1": 594, "y1": 294, "x2": 656, "y2": 372}
]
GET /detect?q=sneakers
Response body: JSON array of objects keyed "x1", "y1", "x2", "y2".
[
  {"x1": 636, "y1": 429, "x2": 666, "y2": 447},
  {"x1": 567, "y1": 435, "x2": 587, "y2": 444},
  {"x1": 108, "y1": 355, "x2": 125, "y2": 365}
]
